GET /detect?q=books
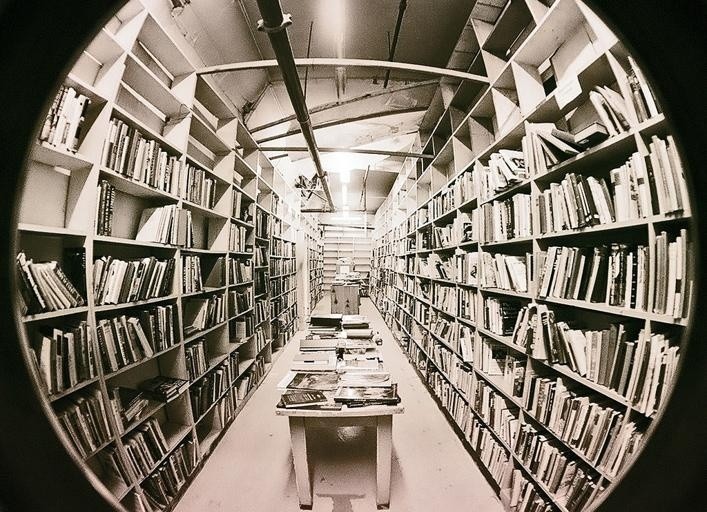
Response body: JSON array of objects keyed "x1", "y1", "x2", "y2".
[
  {"x1": 404, "y1": 293, "x2": 413, "y2": 314},
  {"x1": 269, "y1": 274, "x2": 295, "y2": 298},
  {"x1": 40, "y1": 81, "x2": 90, "y2": 152},
  {"x1": 514, "y1": 411, "x2": 607, "y2": 512},
  {"x1": 449, "y1": 386, "x2": 471, "y2": 439},
  {"x1": 457, "y1": 247, "x2": 478, "y2": 286},
  {"x1": 58, "y1": 389, "x2": 111, "y2": 458},
  {"x1": 470, "y1": 414, "x2": 508, "y2": 489},
  {"x1": 536, "y1": 229, "x2": 696, "y2": 320},
  {"x1": 427, "y1": 333, "x2": 451, "y2": 377},
  {"x1": 255, "y1": 238, "x2": 268, "y2": 267},
  {"x1": 231, "y1": 190, "x2": 254, "y2": 222},
  {"x1": 205, "y1": 355, "x2": 266, "y2": 430},
  {"x1": 457, "y1": 209, "x2": 476, "y2": 244},
  {"x1": 273, "y1": 239, "x2": 296, "y2": 258},
  {"x1": 97, "y1": 179, "x2": 115, "y2": 237},
  {"x1": 239, "y1": 326, "x2": 267, "y2": 358},
  {"x1": 479, "y1": 247, "x2": 533, "y2": 292},
  {"x1": 456, "y1": 288, "x2": 478, "y2": 324},
  {"x1": 416, "y1": 200, "x2": 431, "y2": 227},
  {"x1": 97, "y1": 305, "x2": 179, "y2": 373},
  {"x1": 455, "y1": 322, "x2": 476, "y2": 366},
  {"x1": 411, "y1": 340, "x2": 427, "y2": 377},
  {"x1": 273, "y1": 317, "x2": 297, "y2": 347},
  {"x1": 273, "y1": 304, "x2": 296, "y2": 332},
  {"x1": 184, "y1": 293, "x2": 225, "y2": 335},
  {"x1": 432, "y1": 185, "x2": 455, "y2": 219},
  {"x1": 433, "y1": 218, "x2": 460, "y2": 249},
  {"x1": 474, "y1": 379, "x2": 519, "y2": 452},
  {"x1": 451, "y1": 356, "x2": 475, "y2": 403},
  {"x1": 255, "y1": 270, "x2": 269, "y2": 294},
  {"x1": 271, "y1": 215, "x2": 295, "y2": 242},
  {"x1": 412, "y1": 319, "x2": 427, "y2": 351},
  {"x1": 96, "y1": 414, "x2": 170, "y2": 487},
  {"x1": 414, "y1": 298, "x2": 428, "y2": 327},
  {"x1": 15, "y1": 252, "x2": 84, "y2": 312},
  {"x1": 255, "y1": 299, "x2": 272, "y2": 325},
  {"x1": 432, "y1": 247, "x2": 457, "y2": 281},
  {"x1": 479, "y1": 294, "x2": 536, "y2": 354},
  {"x1": 532, "y1": 151, "x2": 656, "y2": 234},
  {"x1": 455, "y1": 168, "x2": 476, "y2": 203},
  {"x1": 430, "y1": 279, "x2": 455, "y2": 314},
  {"x1": 624, "y1": 55, "x2": 662, "y2": 123},
  {"x1": 400, "y1": 326, "x2": 410, "y2": 355},
  {"x1": 228, "y1": 257, "x2": 253, "y2": 283},
  {"x1": 407, "y1": 211, "x2": 416, "y2": 233},
  {"x1": 139, "y1": 375, "x2": 188, "y2": 402},
  {"x1": 130, "y1": 439, "x2": 197, "y2": 512},
  {"x1": 405, "y1": 253, "x2": 415, "y2": 275},
  {"x1": 638, "y1": 129, "x2": 690, "y2": 216},
  {"x1": 94, "y1": 255, "x2": 176, "y2": 307},
  {"x1": 524, "y1": 82, "x2": 629, "y2": 177},
  {"x1": 230, "y1": 222, "x2": 247, "y2": 253},
  {"x1": 257, "y1": 208, "x2": 270, "y2": 238},
  {"x1": 28, "y1": 321, "x2": 94, "y2": 394},
  {"x1": 415, "y1": 277, "x2": 430, "y2": 302},
  {"x1": 183, "y1": 163, "x2": 217, "y2": 211},
  {"x1": 526, "y1": 360, "x2": 644, "y2": 476},
  {"x1": 424, "y1": 358, "x2": 449, "y2": 408},
  {"x1": 269, "y1": 289, "x2": 297, "y2": 319},
  {"x1": 428, "y1": 306, "x2": 453, "y2": 348},
  {"x1": 417, "y1": 253, "x2": 431, "y2": 278},
  {"x1": 269, "y1": 257, "x2": 296, "y2": 275},
  {"x1": 415, "y1": 227, "x2": 432, "y2": 251},
  {"x1": 135, "y1": 203, "x2": 196, "y2": 249},
  {"x1": 403, "y1": 310, "x2": 411, "y2": 333},
  {"x1": 101, "y1": 116, "x2": 180, "y2": 197},
  {"x1": 276, "y1": 314, "x2": 401, "y2": 410},
  {"x1": 186, "y1": 338, "x2": 209, "y2": 382},
  {"x1": 478, "y1": 334, "x2": 524, "y2": 399},
  {"x1": 108, "y1": 381, "x2": 149, "y2": 436},
  {"x1": 475, "y1": 135, "x2": 535, "y2": 204},
  {"x1": 182, "y1": 255, "x2": 203, "y2": 295},
  {"x1": 508, "y1": 461, "x2": 562, "y2": 512},
  {"x1": 231, "y1": 312, "x2": 254, "y2": 340},
  {"x1": 230, "y1": 286, "x2": 253, "y2": 315},
  {"x1": 475, "y1": 192, "x2": 534, "y2": 244},
  {"x1": 406, "y1": 233, "x2": 417, "y2": 253},
  {"x1": 404, "y1": 275, "x2": 415, "y2": 296},
  {"x1": 201, "y1": 255, "x2": 225, "y2": 288},
  {"x1": 532, "y1": 302, "x2": 680, "y2": 419}
]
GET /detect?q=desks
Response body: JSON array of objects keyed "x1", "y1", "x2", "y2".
[
  {"x1": 277, "y1": 314, "x2": 403, "y2": 509},
  {"x1": 331, "y1": 281, "x2": 360, "y2": 315}
]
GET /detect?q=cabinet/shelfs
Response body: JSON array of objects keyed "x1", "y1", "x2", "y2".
[
  {"x1": 368, "y1": 0, "x2": 695, "y2": 512},
  {"x1": 14, "y1": 1, "x2": 326, "y2": 512},
  {"x1": 323, "y1": 236, "x2": 372, "y2": 297}
]
